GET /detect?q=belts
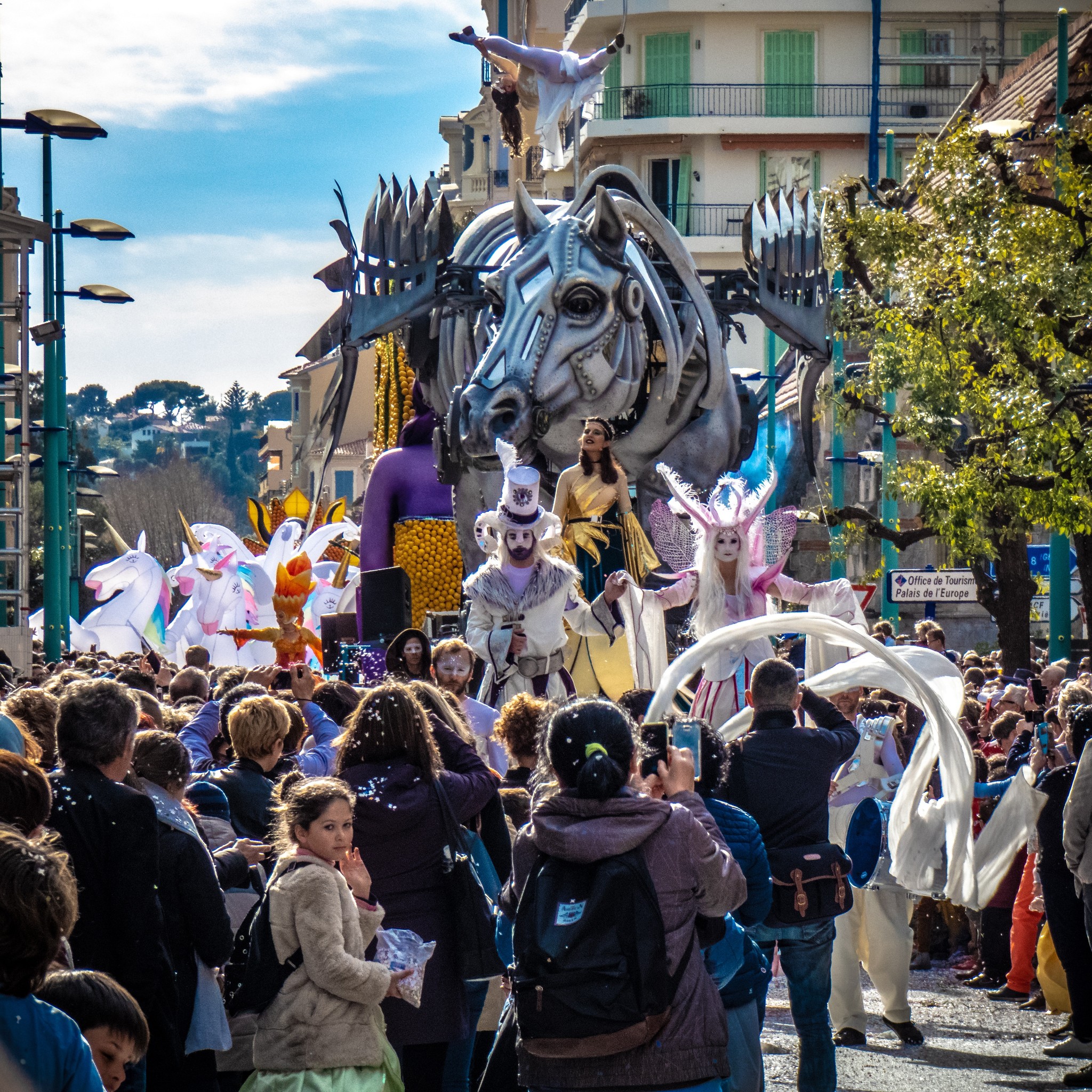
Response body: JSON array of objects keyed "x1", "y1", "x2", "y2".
[
  {"x1": 567, "y1": 515, "x2": 611, "y2": 524},
  {"x1": 517, "y1": 648, "x2": 564, "y2": 679}
]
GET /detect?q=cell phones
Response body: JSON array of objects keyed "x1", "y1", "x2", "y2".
[
  {"x1": 157, "y1": 688, "x2": 163, "y2": 703},
  {"x1": 147, "y1": 649, "x2": 161, "y2": 674},
  {"x1": 887, "y1": 704, "x2": 900, "y2": 713},
  {"x1": 983, "y1": 698, "x2": 992, "y2": 720},
  {"x1": 671, "y1": 721, "x2": 703, "y2": 780},
  {"x1": 271, "y1": 669, "x2": 303, "y2": 690},
  {"x1": 1028, "y1": 679, "x2": 1045, "y2": 706},
  {"x1": 932, "y1": 770, "x2": 943, "y2": 800},
  {"x1": 1024, "y1": 710, "x2": 1050, "y2": 754},
  {"x1": 639, "y1": 721, "x2": 667, "y2": 780}
]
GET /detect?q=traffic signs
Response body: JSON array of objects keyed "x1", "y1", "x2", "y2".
[
  {"x1": 989, "y1": 544, "x2": 1079, "y2": 578},
  {"x1": 993, "y1": 578, "x2": 1085, "y2": 595},
  {"x1": 990, "y1": 595, "x2": 1083, "y2": 624}
]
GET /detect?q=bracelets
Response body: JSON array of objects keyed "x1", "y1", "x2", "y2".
[{"x1": 297, "y1": 699, "x2": 312, "y2": 701}]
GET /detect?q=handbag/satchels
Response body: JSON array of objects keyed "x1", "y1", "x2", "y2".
[
  {"x1": 433, "y1": 774, "x2": 508, "y2": 982},
  {"x1": 766, "y1": 843, "x2": 854, "y2": 930}
]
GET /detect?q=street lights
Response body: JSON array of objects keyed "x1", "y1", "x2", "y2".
[
  {"x1": 42, "y1": 128, "x2": 136, "y2": 671},
  {"x1": 729, "y1": 369, "x2": 781, "y2": 648}
]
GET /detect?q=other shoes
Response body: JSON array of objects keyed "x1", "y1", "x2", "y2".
[
  {"x1": 955, "y1": 960, "x2": 984, "y2": 980},
  {"x1": 1063, "y1": 1062, "x2": 1092, "y2": 1089},
  {"x1": 1018, "y1": 989, "x2": 1046, "y2": 1011},
  {"x1": 962, "y1": 971, "x2": 1005, "y2": 988},
  {"x1": 986, "y1": 984, "x2": 1030, "y2": 1002},
  {"x1": 832, "y1": 1027, "x2": 867, "y2": 1047},
  {"x1": 1043, "y1": 1035, "x2": 1092, "y2": 1058},
  {"x1": 1046, "y1": 1015, "x2": 1073, "y2": 1040},
  {"x1": 882, "y1": 1015, "x2": 924, "y2": 1045},
  {"x1": 909, "y1": 953, "x2": 931, "y2": 970}
]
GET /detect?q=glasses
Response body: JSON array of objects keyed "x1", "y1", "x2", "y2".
[
  {"x1": 927, "y1": 639, "x2": 937, "y2": 644},
  {"x1": 998, "y1": 698, "x2": 1015, "y2": 705}
]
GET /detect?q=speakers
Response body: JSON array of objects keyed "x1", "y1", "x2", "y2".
[
  {"x1": 320, "y1": 612, "x2": 361, "y2": 675},
  {"x1": 363, "y1": 566, "x2": 411, "y2": 650}
]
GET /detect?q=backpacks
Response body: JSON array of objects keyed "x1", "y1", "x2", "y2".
[
  {"x1": 511, "y1": 848, "x2": 694, "y2": 1058},
  {"x1": 223, "y1": 861, "x2": 314, "y2": 1017}
]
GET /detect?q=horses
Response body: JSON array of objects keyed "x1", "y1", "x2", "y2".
[
  {"x1": 416, "y1": 179, "x2": 741, "y2": 665},
  {"x1": 29, "y1": 517, "x2": 175, "y2": 667},
  {"x1": 165, "y1": 509, "x2": 364, "y2": 683}
]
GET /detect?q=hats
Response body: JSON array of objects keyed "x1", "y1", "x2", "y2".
[
  {"x1": 999, "y1": 669, "x2": 1035, "y2": 687},
  {"x1": 184, "y1": 781, "x2": 230, "y2": 822},
  {"x1": 498, "y1": 466, "x2": 545, "y2": 530}
]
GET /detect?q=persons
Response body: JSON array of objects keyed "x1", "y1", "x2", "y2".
[
  {"x1": 553, "y1": 417, "x2": 661, "y2": 698},
  {"x1": 462, "y1": 509, "x2": 627, "y2": 715},
  {"x1": 0, "y1": 621, "x2": 1092, "y2": 1092},
  {"x1": 615, "y1": 519, "x2": 855, "y2": 731},
  {"x1": 216, "y1": 603, "x2": 326, "y2": 685},
  {"x1": 447, "y1": 29, "x2": 626, "y2": 172}
]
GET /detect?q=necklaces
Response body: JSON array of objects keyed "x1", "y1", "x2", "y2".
[
  {"x1": 590, "y1": 458, "x2": 601, "y2": 463},
  {"x1": 284, "y1": 628, "x2": 296, "y2": 633}
]
